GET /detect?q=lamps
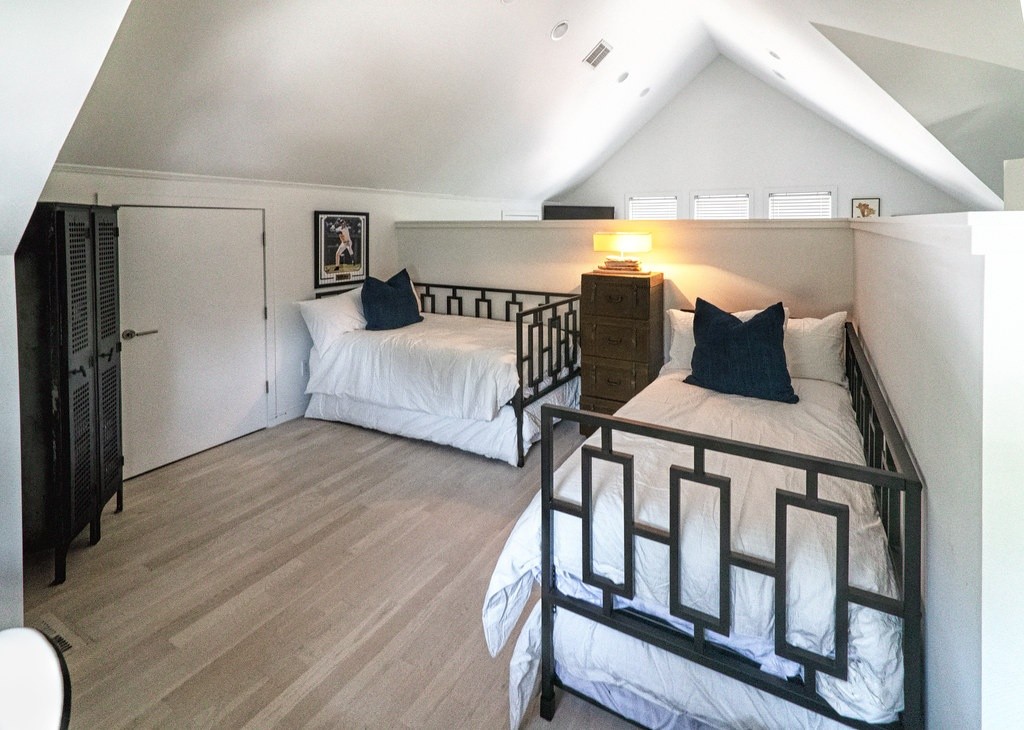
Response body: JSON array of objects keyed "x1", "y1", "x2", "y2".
[{"x1": 593, "y1": 232, "x2": 651, "y2": 274}]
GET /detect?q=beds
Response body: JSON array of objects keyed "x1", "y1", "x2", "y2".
[
  {"x1": 301, "y1": 282, "x2": 582, "y2": 469},
  {"x1": 483, "y1": 306, "x2": 926, "y2": 730}
]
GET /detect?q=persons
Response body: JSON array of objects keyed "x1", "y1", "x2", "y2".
[{"x1": 330, "y1": 217, "x2": 355, "y2": 271}]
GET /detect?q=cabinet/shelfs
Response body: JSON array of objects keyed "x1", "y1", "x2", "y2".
[
  {"x1": 14, "y1": 201, "x2": 126, "y2": 585},
  {"x1": 580, "y1": 270, "x2": 665, "y2": 438}
]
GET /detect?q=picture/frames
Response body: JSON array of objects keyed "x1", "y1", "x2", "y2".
[
  {"x1": 313, "y1": 210, "x2": 370, "y2": 289},
  {"x1": 852, "y1": 198, "x2": 881, "y2": 219}
]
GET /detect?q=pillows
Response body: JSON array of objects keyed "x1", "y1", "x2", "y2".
[
  {"x1": 293, "y1": 268, "x2": 423, "y2": 358},
  {"x1": 659, "y1": 297, "x2": 848, "y2": 406}
]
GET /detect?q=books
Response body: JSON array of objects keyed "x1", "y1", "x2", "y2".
[{"x1": 592, "y1": 257, "x2": 651, "y2": 275}]
